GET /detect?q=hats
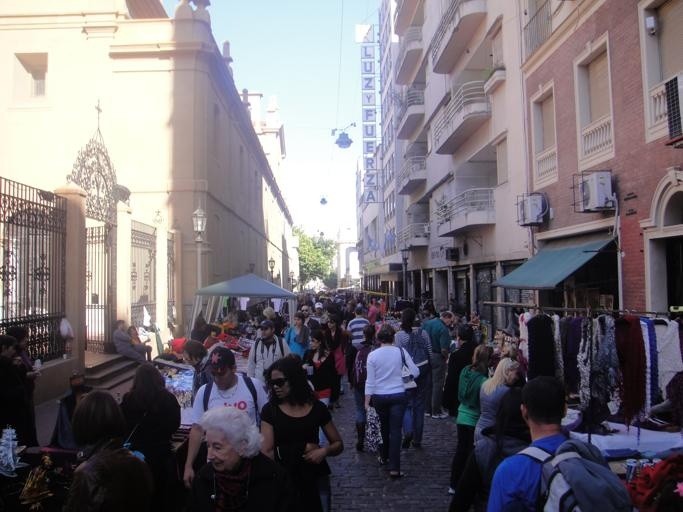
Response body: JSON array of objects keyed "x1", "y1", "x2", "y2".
[
  {"x1": 208, "y1": 346, "x2": 234, "y2": 369},
  {"x1": 258, "y1": 320, "x2": 274, "y2": 328},
  {"x1": 315, "y1": 302, "x2": 323, "y2": 308}
]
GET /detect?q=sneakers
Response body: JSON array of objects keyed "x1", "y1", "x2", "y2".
[
  {"x1": 424, "y1": 412, "x2": 431, "y2": 416},
  {"x1": 378, "y1": 457, "x2": 387, "y2": 464},
  {"x1": 402, "y1": 431, "x2": 412, "y2": 449},
  {"x1": 432, "y1": 412, "x2": 448, "y2": 418},
  {"x1": 390, "y1": 471, "x2": 403, "y2": 480}
]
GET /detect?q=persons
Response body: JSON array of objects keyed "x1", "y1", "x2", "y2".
[
  {"x1": 1, "y1": 289, "x2": 632, "y2": 511},
  {"x1": 631, "y1": 371, "x2": 683, "y2": 426}
]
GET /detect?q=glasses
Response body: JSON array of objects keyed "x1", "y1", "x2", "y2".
[{"x1": 271, "y1": 377, "x2": 289, "y2": 387}]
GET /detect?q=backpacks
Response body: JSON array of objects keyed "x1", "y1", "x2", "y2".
[
  {"x1": 353, "y1": 343, "x2": 377, "y2": 391},
  {"x1": 404, "y1": 329, "x2": 428, "y2": 367},
  {"x1": 517, "y1": 447, "x2": 590, "y2": 512}
]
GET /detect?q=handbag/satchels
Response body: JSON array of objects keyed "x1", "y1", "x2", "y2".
[{"x1": 401, "y1": 364, "x2": 417, "y2": 390}]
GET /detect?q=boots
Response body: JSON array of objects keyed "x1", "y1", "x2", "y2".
[{"x1": 356, "y1": 421, "x2": 365, "y2": 450}]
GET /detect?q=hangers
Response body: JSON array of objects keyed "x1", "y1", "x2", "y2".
[{"x1": 519, "y1": 306, "x2": 683, "y2": 325}]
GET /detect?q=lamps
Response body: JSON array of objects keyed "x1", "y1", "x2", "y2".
[{"x1": 646, "y1": 16, "x2": 658, "y2": 36}]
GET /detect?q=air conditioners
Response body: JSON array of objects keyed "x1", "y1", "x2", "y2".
[
  {"x1": 579, "y1": 171, "x2": 613, "y2": 211},
  {"x1": 517, "y1": 195, "x2": 543, "y2": 226}
]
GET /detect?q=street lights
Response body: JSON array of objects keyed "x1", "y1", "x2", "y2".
[
  {"x1": 289, "y1": 271, "x2": 294, "y2": 292},
  {"x1": 267, "y1": 255, "x2": 275, "y2": 283},
  {"x1": 190, "y1": 197, "x2": 207, "y2": 314},
  {"x1": 247, "y1": 263, "x2": 255, "y2": 273}
]
